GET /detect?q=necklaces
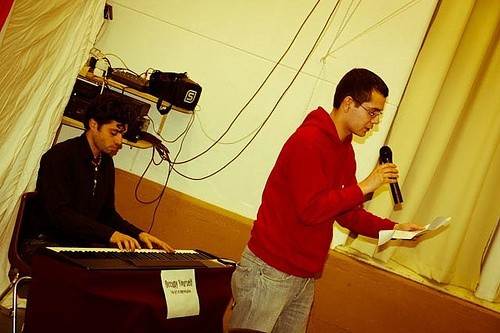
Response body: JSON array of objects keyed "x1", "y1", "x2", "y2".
[{"x1": 92, "y1": 153, "x2": 102, "y2": 166}]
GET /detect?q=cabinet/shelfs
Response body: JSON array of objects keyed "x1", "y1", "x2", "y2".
[{"x1": 61, "y1": 72, "x2": 194, "y2": 149}]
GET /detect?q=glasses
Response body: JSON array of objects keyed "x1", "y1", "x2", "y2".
[{"x1": 352, "y1": 97, "x2": 382, "y2": 118}]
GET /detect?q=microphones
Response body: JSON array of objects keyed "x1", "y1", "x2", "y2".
[{"x1": 380, "y1": 146, "x2": 404, "y2": 204}]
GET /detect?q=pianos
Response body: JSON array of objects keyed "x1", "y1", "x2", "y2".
[{"x1": 39, "y1": 242, "x2": 238, "y2": 270}]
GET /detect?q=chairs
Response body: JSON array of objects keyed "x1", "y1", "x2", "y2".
[{"x1": 0, "y1": 191, "x2": 36, "y2": 333}]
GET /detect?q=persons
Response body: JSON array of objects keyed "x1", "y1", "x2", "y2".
[
  {"x1": 223, "y1": 67, "x2": 429, "y2": 333},
  {"x1": 22, "y1": 93, "x2": 174, "y2": 264}
]
